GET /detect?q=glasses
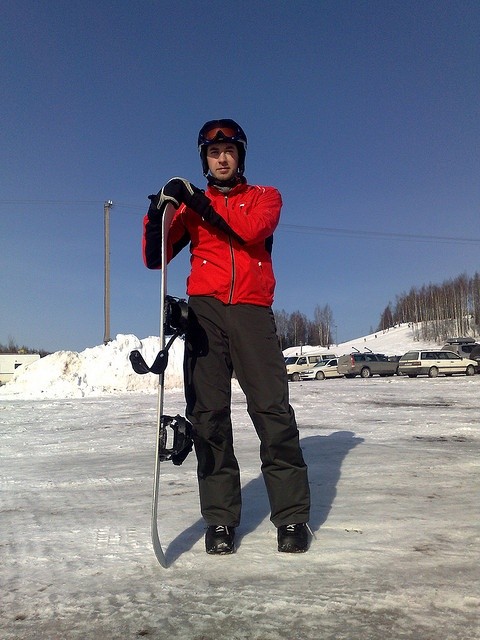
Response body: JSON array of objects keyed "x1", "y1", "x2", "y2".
[{"x1": 203, "y1": 124, "x2": 235, "y2": 140}]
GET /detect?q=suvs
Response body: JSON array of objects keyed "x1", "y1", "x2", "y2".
[
  {"x1": 285, "y1": 354, "x2": 336, "y2": 382},
  {"x1": 440, "y1": 336, "x2": 478, "y2": 358},
  {"x1": 399, "y1": 350, "x2": 478, "y2": 378},
  {"x1": 337, "y1": 347, "x2": 403, "y2": 379}
]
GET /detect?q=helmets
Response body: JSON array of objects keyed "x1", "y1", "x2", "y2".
[{"x1": 197, "y1": 119, "x2": 246, "y2": 189}]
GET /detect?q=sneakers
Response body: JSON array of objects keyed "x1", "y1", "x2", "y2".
[
  {"x1": 205, "y1": 525, "x2": 234, "y2": 554},
  {"x1": 278, "y1": 523, "x2": 318, "y2": 552}
]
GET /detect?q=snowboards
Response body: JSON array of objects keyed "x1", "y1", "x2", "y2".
[{"x1": 149, "y1": 202, "x2": 196, "y2": 569}]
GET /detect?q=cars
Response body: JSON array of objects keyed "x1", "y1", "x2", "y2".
[{"x1": 299, "y1": 358, "x2": 340, "y2": 382}]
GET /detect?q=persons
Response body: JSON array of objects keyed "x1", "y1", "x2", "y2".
[{"x1": 142, "y1": 119, "x2": 311, "y2": 555}]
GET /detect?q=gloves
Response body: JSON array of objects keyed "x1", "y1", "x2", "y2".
[
  {"x1": 149, "y1": 190, "x2": 179, "y2": 215},
  {"x1": 164, "y1": 177, "x2": 211, "y2": 212}
]
